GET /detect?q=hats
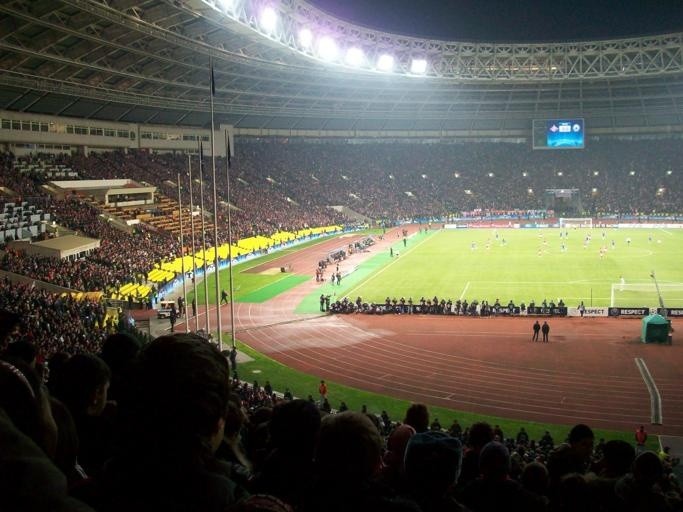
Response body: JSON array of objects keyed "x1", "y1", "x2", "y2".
[{"x1": 384, "y1": 424, "x2": 463, "y2": 478}]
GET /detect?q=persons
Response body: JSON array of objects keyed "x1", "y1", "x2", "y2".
[
  {"x1": 246, "y1": 380, "x2": 682, "y2": 507},
  {"x1": 532, "y1": 321, "x2": 552, "y2": 344},
  {"x1": 319, "y1": 291, "x2": 589, "y2": 318},
  {"x1": 312, "y1": 261, "x2": 343, "y2": 288},
  {"x1": 1, "y1": 140, "x2": 246, "y2": 512},
  {"x1": 246, "y1": 137, "x2": 682, "y2": 259}
]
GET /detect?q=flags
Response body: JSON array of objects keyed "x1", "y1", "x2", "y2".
[{"x1": 211, "y1": 67, "x2": 216, "y2": 97}]
